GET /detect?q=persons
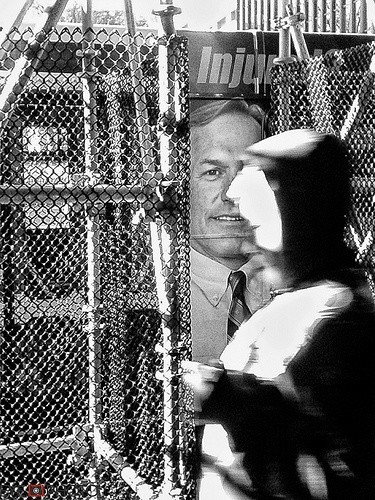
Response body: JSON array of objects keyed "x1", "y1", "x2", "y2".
[
  {"x1": 190, "y1": 93, "x2": 273, "y2": 368},
  {"x1": 180, "y1": 128, "x2": 375, "y2": 500}
]
